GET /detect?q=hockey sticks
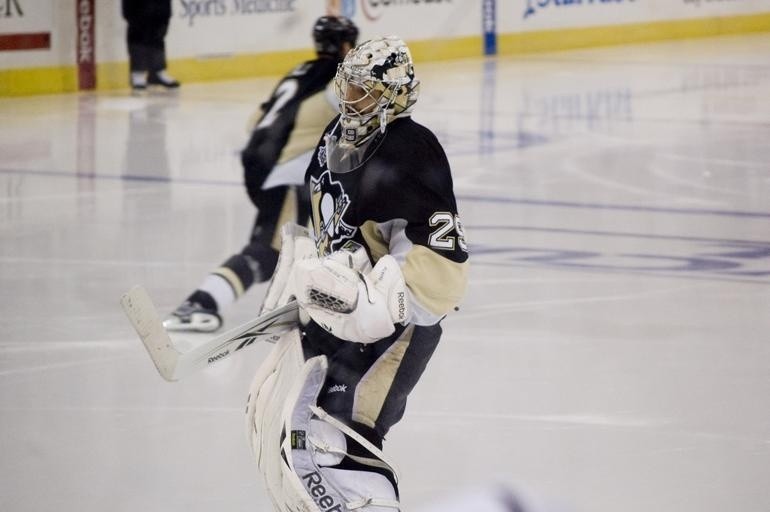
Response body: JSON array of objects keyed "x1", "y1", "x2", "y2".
[{"x1": 121, "y1": 284, "x2": 299, "y2": 382}]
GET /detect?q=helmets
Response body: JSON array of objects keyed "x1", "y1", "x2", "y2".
[
  {"x1": 313, "y1": 16, "x2": 359, "y2": 55},
  {"x1": 333, "y1": 35, "x2": 419, "y2": 135}
]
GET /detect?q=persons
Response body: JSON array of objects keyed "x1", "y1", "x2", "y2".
[
  {"x1": 122, "y1": 0, "x2": 180, "y2": 89},
  {"x1": 170, "y1": 16, "x2": 360, "y2": 333},
  {"x1": 244, "y1": 38, "x2": 469, "y2": 512}
]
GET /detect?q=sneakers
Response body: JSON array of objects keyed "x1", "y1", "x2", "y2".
[
  {"x1": 162, "y1": 301, "x2": 222, "y2": 332},
  {"x1": 147, "y1": 71, "x2": 179, "y2": 87},
  {"x1": 129, "y1": 72, "x2": 149, "y2": 88}
]
